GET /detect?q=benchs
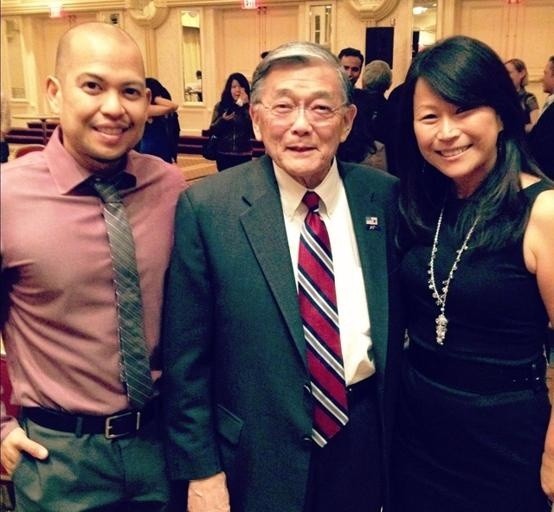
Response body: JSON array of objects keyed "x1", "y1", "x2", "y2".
[{"x1": 176, "y1": 135, "x2": 266, "y2": 160}]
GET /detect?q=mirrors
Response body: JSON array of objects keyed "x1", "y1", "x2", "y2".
[
  {"x1": 180, "y1": 9, "x2": 205, "y2": 102},
  {"x1": 412, "y1": 0, "x2": 436, "y2": 64}
]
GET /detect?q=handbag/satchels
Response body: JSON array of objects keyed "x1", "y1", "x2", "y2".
[{"x1": 203, "y1": 138, "x2": 217, "y2": 160}]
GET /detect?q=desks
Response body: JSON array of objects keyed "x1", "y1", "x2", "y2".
[{"x1": 12, "y1": 114, "x2": 60, "y2": 145}]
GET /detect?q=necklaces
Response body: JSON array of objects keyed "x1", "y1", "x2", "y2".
[{"x1": 427, "y1": 205, "x2": 481, "y2": 346}]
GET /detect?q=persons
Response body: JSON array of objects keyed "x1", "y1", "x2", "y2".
[
  {"x1": 141, "y1": 76, "x2": 180, "y2": 164},
  {"x1": 0, "y1": 21, "x2": 187, "y2": 511},
  {"x1": 156, "y1": 44, "x2": 401, "y2": 511},
  {"x1": 525, "y1": 55, "x2": 554, "y2": 181},
  {"x1": 191, "y1": 70, "x2": 203, "y2": 102},
  {"x1": 505, "y1": 58, "x2": 539, "y2": 132},
  {"x1": 332, "y1": 48, "x2": 415, "y2": 179},
  {"x1": 394, "y1": 34, "x2": 554, "y2": 512},
  {"x1": 209, "y1": 73, "x2": 253, "y2": 171}
]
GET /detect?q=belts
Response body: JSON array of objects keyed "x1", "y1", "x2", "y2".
[{"x1": 25, "y1": 406, "x2": 158, "y2": 440}]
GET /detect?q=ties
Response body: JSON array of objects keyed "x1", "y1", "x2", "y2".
[
  {"x1": 92, "y1": 174, "x2": 154, "y2": 411},
  {"x1": 298, "y1": 192, "x2": 350, "y2": 448}
]
